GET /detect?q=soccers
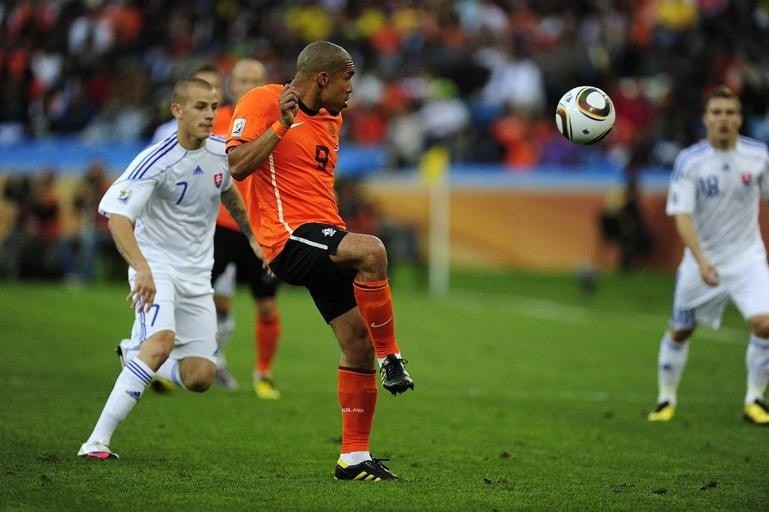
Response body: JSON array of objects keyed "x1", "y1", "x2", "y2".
[{"x1": 555, "y1": 86, "x2": 617, "y2": 146}]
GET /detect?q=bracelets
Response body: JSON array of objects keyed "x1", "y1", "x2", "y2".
[{"x1": 272, "y1": 120, "x2": 286, "y2": 141}]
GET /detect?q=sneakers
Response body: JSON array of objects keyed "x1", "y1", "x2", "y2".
[
  {"x1": 217, "y1": 367, "x2": 239, "y2": 393},
  {"x1": 251, "y1": 373, "x2": 281, "y2": 400},
  {"x1": 334, "y1": 453, "x2": 400, "y2": 482},
  {"x1": 77, "y1": 441, "x2": 120, "y2": 460},
  {"x1": 115, "y1": 338, "x2": 131, "y2": 370},
  {"x1": 743, "y1": 400, "x2": 769, "y2": 427},
  {"x1": 647, "y1": 402, "x2": 675, "y2": 422},
  {"x1": 151, "y1": 376, "x2": 175, "y2": 393},
  {"x1": 380, "y1": 355, "x2": 414, "y2": 396}
]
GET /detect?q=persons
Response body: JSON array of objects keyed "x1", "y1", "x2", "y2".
[
  {"x1": 647, "y1": 85, "x2": 768, "y2": 426},
  {"x1": 197, "y1": 58, "x2": 283, "y2": 399},
  {"x1": 331, "y1": 171, "x2": 409, "y2": 273},
  {"x1": 599, "y1": 161, "x2": 650, "y2": 269},
  {"x1": 17, "y1": 168, "x2": 59, "y2": 281},
  {"x1": 154, "y1": 58, "x2": 241, "y2": 391},
  {"x1": 1, "y1": 1, "x2": 768, "y2": 158},
  {"x1": 85, "y1": 162, "x2": 130, "y2": 281},
  {"x1": 224, "y1": 40, "x2": 419, "y2": 484},
  {"x1": 77, "y1": 77, "x2": 272, "y2": 460}
]
